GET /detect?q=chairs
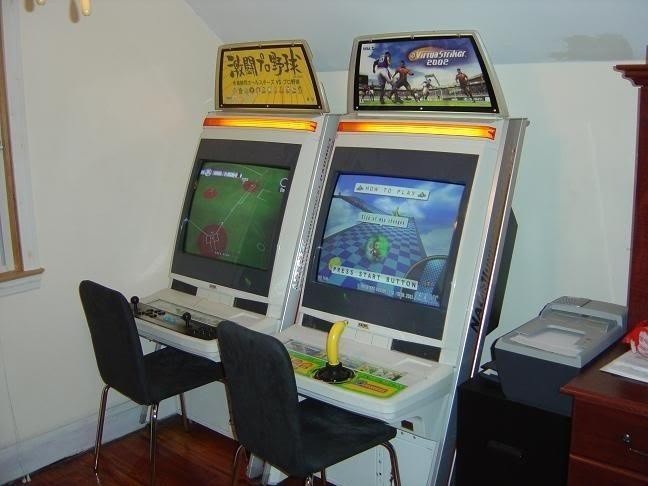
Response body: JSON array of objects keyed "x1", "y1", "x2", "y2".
[
  {"x1": 78, "y1": 279, "x2": 226, "y2": 485},
  {"x1": 216, "y1": 318, "x2": 401, "y2": 484}
]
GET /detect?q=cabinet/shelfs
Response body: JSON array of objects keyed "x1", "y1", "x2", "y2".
[
  {"x1": 457, "y1": 376, "x2": 572, "y2": 486},
  {"x1": 558, "y1": 337, "x2": 648, "y2": 486}
]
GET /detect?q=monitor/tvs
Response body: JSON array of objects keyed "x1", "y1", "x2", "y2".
[
  {"x1": 176, "y1": 157, "x2": 293, "y2": 272},
  {"x1": 314, "y1": 170, "x2": 470, "y2": 312}
]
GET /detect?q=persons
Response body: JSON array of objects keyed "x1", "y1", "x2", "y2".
[{"x1": 359, "y1": 52, "x2": 475, "y2": 104}]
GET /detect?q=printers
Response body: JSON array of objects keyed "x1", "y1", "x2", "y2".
[{"x1": 492, "y1": 297, "x2": 629, "y2": 416}]
